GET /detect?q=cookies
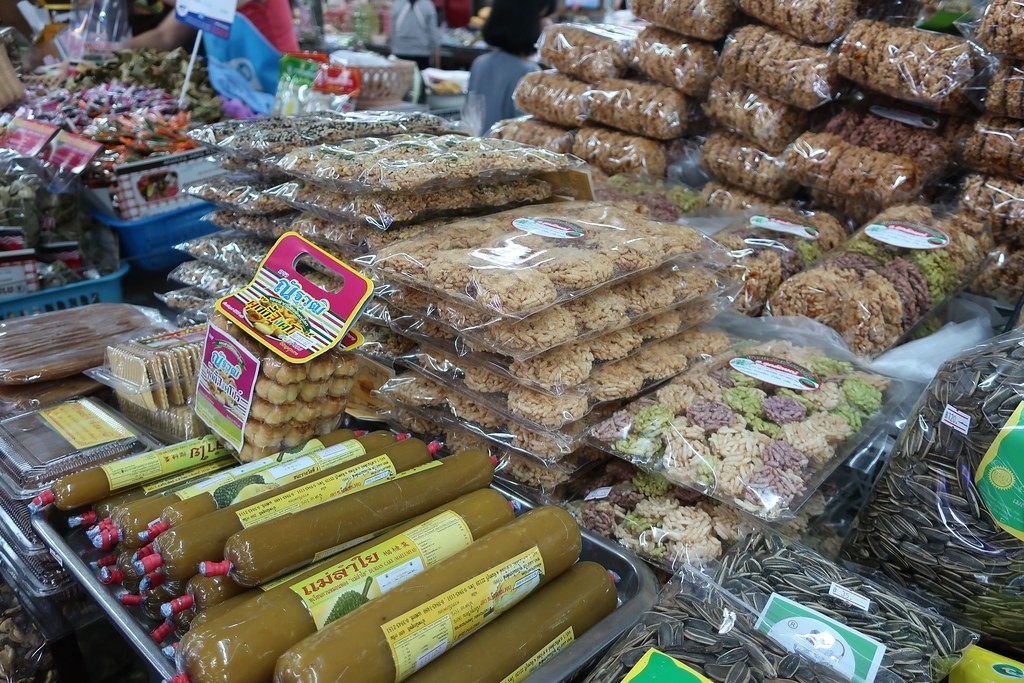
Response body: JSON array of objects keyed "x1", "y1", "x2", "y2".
[{"x1": 106, "y1": 108, "x2": 888, "y2": 566}]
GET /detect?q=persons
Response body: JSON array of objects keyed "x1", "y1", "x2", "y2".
[
  {"x1": 464, "y1": 0, "x2": 544, "y2": 137},
  {"x1": 89, "y1": 0, "x2": 303, "y2": 119},
  {"x1": 388, "y1": 0, "x2": 443, "y2": 104}
]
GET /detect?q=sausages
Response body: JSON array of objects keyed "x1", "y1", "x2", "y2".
[{"x1": 51, "y1": 430, "x2": 616, "y2": 682}]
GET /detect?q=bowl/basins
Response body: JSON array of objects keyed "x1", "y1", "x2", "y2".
[{"x1": 426, "y1": 92, "x2": 467, "y2": 109}]
